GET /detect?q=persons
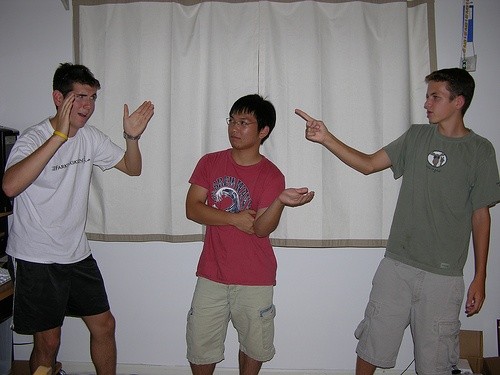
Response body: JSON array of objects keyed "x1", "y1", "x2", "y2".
[
  {"x1": 295, "y1": 67, "x2": 500, "y2": 375},
  {"x1": 2, "y1": 64, "x2": 154, "y2": 375},
  {"x1": 186, "y1": 94, "x2": 314, "y2": 375}
]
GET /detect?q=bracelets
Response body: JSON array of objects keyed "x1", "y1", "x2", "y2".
[
  {"x1": 53, "y1": 131, "x2": 68, "y2": 140},
  {"x1": 123, "y1": 131, "x2": 141, "y2": 140}
]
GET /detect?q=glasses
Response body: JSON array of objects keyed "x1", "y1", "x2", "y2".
[{"x1": 226, "y1": 116, "x2": 257, "y2": 127}]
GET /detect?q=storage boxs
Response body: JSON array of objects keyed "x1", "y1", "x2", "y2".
[{"x1": 458, "y1": 330, "x2": 483, "y2": 374}]
